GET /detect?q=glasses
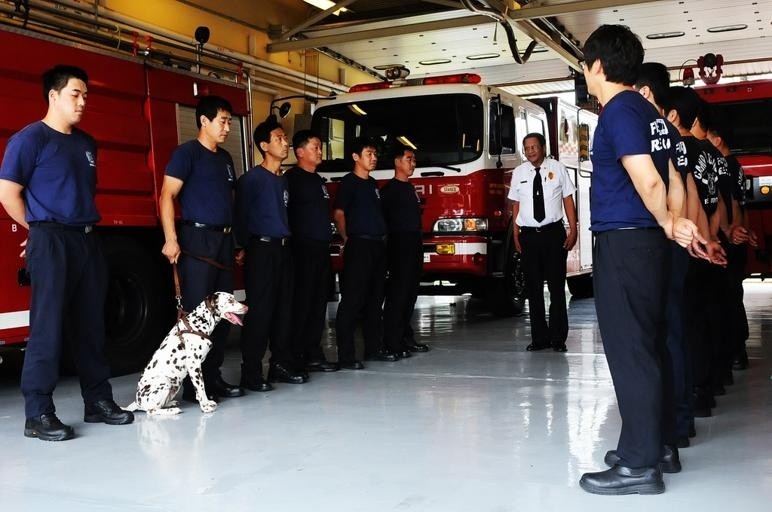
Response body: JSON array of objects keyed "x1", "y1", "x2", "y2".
[
  {"x1": 577, "y1": 59, "x2": 591, "y2": 69},
  {"x1": 522, "y1": 146, "x2": 540, "y2": 154}
]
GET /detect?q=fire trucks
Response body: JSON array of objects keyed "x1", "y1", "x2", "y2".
[
  {"x1": 674, "y1": 51, "x2": 772, "y2": 283},
  {"x1": 0, "y1": 3, "x2": 254, "y2": 374},
  {"x1": 265, "y1": 72, "x2": 600, "y2": 317}
]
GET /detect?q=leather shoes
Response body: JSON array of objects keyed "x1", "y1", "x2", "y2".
[
  {"x1": 24, "y1": 412, "x2": 74, "y2": 441},
  {"x1": 554, "y1": 341, "x2": 566, "y2": 352},
  {"x1": 182, "y1": 339, "x2": 430, "y2": 404},
  {"x1": 84, "y1": 398, "x2": 135, "y2": 426},
  {"x1": 527, "y1": 343, "x2": 540, "y2": 351},
  {"x1": 580, "y1": 344, "x2": 749, "y2": 494}
]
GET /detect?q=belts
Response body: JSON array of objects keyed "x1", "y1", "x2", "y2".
[
  {"x1": 617, "y1": 226, "x2": 643, "y2": 231},
  {"x1": 521, "y1": 225, "x2": 556, "y2": 233},
  {"x1": 30, "y1": 220, "x2": 96, "y2": 235},
  {"x1": 184, "y1": 220, "x2": 232, "y2": 234},
  {"x1": 260, "y1": 233, "x2": 288, "y2": 246}
]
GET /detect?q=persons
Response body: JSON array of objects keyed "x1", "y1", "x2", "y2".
[
  {"x1": 332, "y1": 135, "x2": 400, "y2": 371},
  {"x1": 379, "y1": 145, "x2": 431, "y2": 359},
  {"x1": 579, "y1": 23, "x2": 756, "y2": 495},
  {"x1": 507, "y1": 133, "x2": 577, "y2": 352},
  {"x1": 234, "y1": 120, "x2": 307, "y2": 392},
  {"x1": 283, "y1": 129, "x2": 341, "y2": 380},
  {"x1": 1, "y1": 64, "x2": 135, "y2": 442},
  {"x1": 158, "y1": 94, "x2": 245, "y2": 404}
]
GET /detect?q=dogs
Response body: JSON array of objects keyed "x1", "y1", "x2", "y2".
[{"x1": 119, "y1": 291, "x2": 249, "y2": 416}]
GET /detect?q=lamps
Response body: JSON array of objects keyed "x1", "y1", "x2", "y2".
[{"x1": 272, "y1": 102, "x2": 292, "y2": 119}]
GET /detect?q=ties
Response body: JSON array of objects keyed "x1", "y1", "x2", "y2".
[{"x1": 533, "y1": 166, "x2": 545, "y2": 223}]
